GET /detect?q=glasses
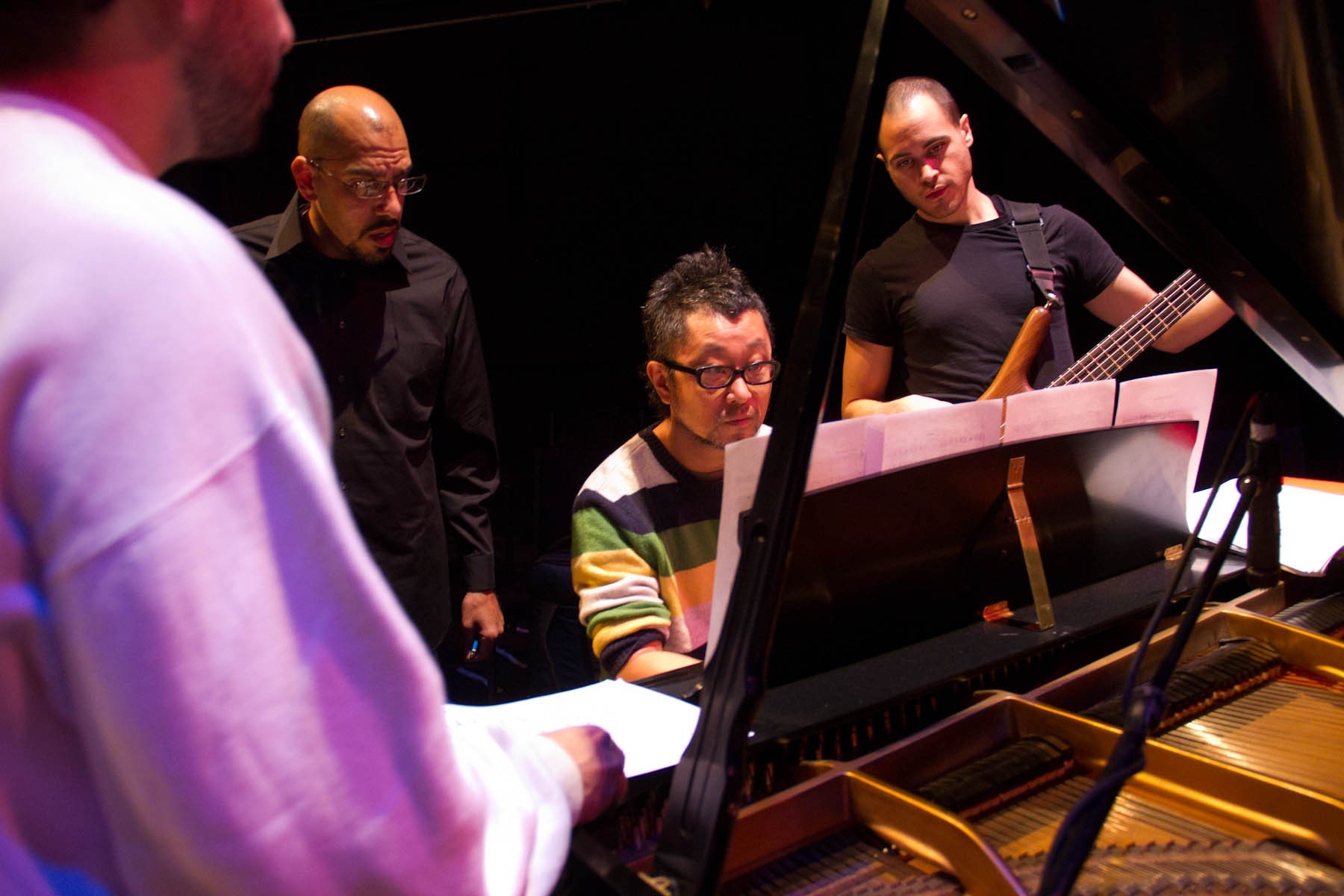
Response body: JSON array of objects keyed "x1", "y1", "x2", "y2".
[
  {"x1": 658, "y1": 358, "x2": 781, "y2": 389},
  {"x1": 308, "y1": 159, "x2": 427, "y2": 199}
]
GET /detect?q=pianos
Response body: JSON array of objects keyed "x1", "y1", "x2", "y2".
[{"x1": 559, "y1": 3, "x2": 1341, "y2": 893}]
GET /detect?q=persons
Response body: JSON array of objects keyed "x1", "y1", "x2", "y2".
[
  {"x1": 842, "y1": 73, "x2": 1238, "y2": 420},
  {"x1": 226, "y1": 83, "x2": 504, "y2": 704},
  {"x1": 0, "y1": 1, "x2": 632, "y2": 896},
  {"x1": 570, "y1": 249, "x2": 777, "y2": 681}
]
